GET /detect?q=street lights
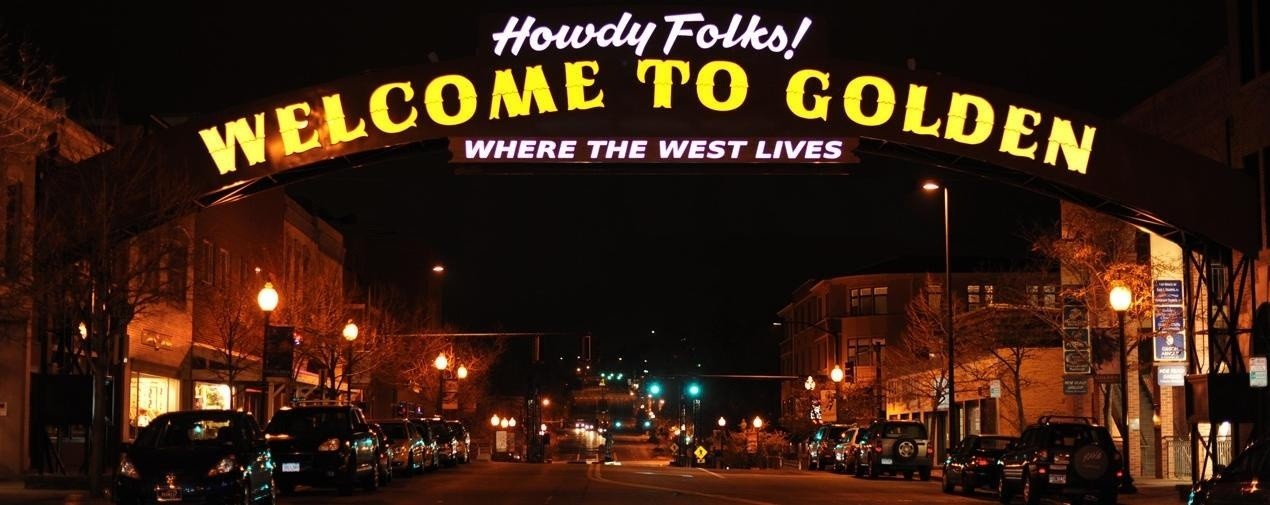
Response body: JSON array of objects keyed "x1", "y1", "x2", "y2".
[
  {"x1": 752, "y1": 415, "x2": 764, "y2": 452},
  {"x1": 1108, "y1": 279, "x2": 1140, "y2": 495},
  {"x1": 253, "y1": 281, "x2": 281, "y2": 429},
  {"x1": 716, "y1": 417, "x2": 728, "y2": 470},
  {"x1": 769, "y1": 318, "x2": 842, "y2": 421},
  {"x1": 919, "y1": 176, "x2": 961, "y2": 453},
  {"x1": 340, "y1": 317, "x2": 362, "y2": 410},
  {"x1": 435, "y1": 349, "x2": 468, "y2": 417}
]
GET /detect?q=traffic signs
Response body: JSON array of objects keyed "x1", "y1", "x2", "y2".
[{"x1": 1094, "y1": 374, "x2": 1121, "y2": 384}]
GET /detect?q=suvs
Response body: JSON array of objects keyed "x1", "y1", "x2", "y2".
[
  {"x1": 806, "y1": 420, "x2": 859, "y2": 471},
  {"x1": 994, "y1": 413, "x2": 1120, "y2": 505},
  {"x1": 851, "y1": 417, "x2": 936, "y2": 483}
]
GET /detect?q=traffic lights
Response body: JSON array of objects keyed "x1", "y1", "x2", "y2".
[
  {"x1": 640, "y1": 419, "x2": 653, "y2": 433},
  {"x1": 575, "y1": 360, "x2": 583, "y2": 376},
  {"x1": 688, "y1": 376, "x2": 700, "y2": 396},
  {"x1": 613, "y1": 416, "x2": 623, "y2": 433},
  {"x1": 651, "y1": 378, "x2": 662, "y2": 396},
  {"x1": 584, "y1": 359, "x2": 592, "y2": 376}
]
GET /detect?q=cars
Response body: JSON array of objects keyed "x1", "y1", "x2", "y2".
[
  {"x1": 565, "y1": 414, "x2": 609, "y2": 434},
  {"x1": 939, "y1": 428, "x2": 1022, "y2": 497},
  {"x1": 832, "y1": 426, "x2": 870, "y2": 474},
  {"x1": 261, "y1": 401, "x2": 477, "y2": 488},
  {"x1": 507, "y1": 452, "x2": 521, "y2": 462},
  {"x1": 1182, "y1": 434, "x2": 1270, "y2": 505},
  {"x1": 108, "y1": 409, "x2": 280, "y2": 505}
]
